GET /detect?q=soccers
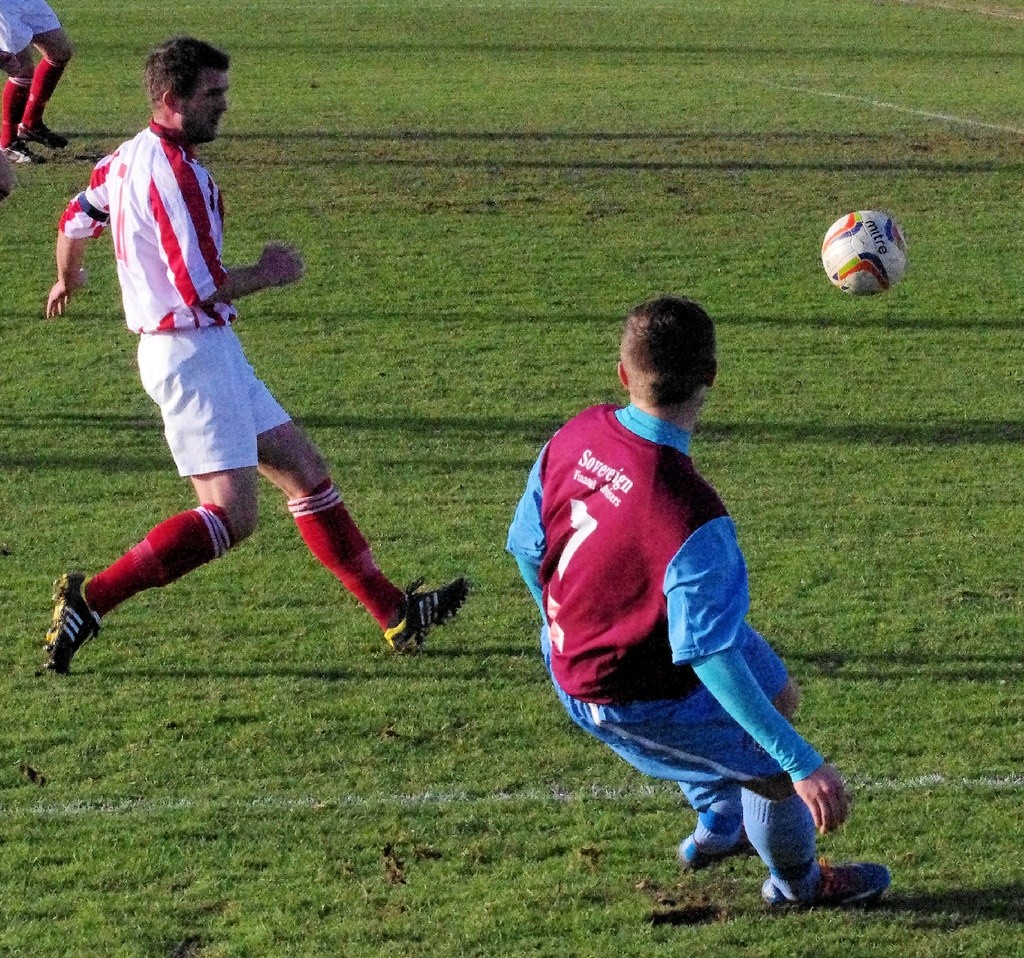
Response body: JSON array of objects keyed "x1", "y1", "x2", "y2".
[{"x1": 821, "y1": 210, "x2": 909, "y2": 297}]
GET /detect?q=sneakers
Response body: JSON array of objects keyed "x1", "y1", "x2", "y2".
[
  {"x1": 41, "y1": 571, "x2": 102, "y2": 675},
  {"x1": 678, "y1": 823, "x2": 756, "y2": 871},
  {"x1": 384, "y1": 576, "x2": 467, "y2": 656},
  {"x1": 1, "y1": 142, "x2": 47, "y2": 165},
  {"x1": 16, "y1": 122, "x2": 69, "y2": 148},
  {"x1": 763, "y1": 864, "x2": 890, "y2": 912}
]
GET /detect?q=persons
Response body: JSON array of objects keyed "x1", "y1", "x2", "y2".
[
  {"x1": 504, "y1": 295, "x2": 892, "y2": 910},
  {"x1": 45, "y1": 39, "x2": 469, "y2": 674},
  {"x1": 0, "y1": 0, "x2": 72, "y2": 165}
]
